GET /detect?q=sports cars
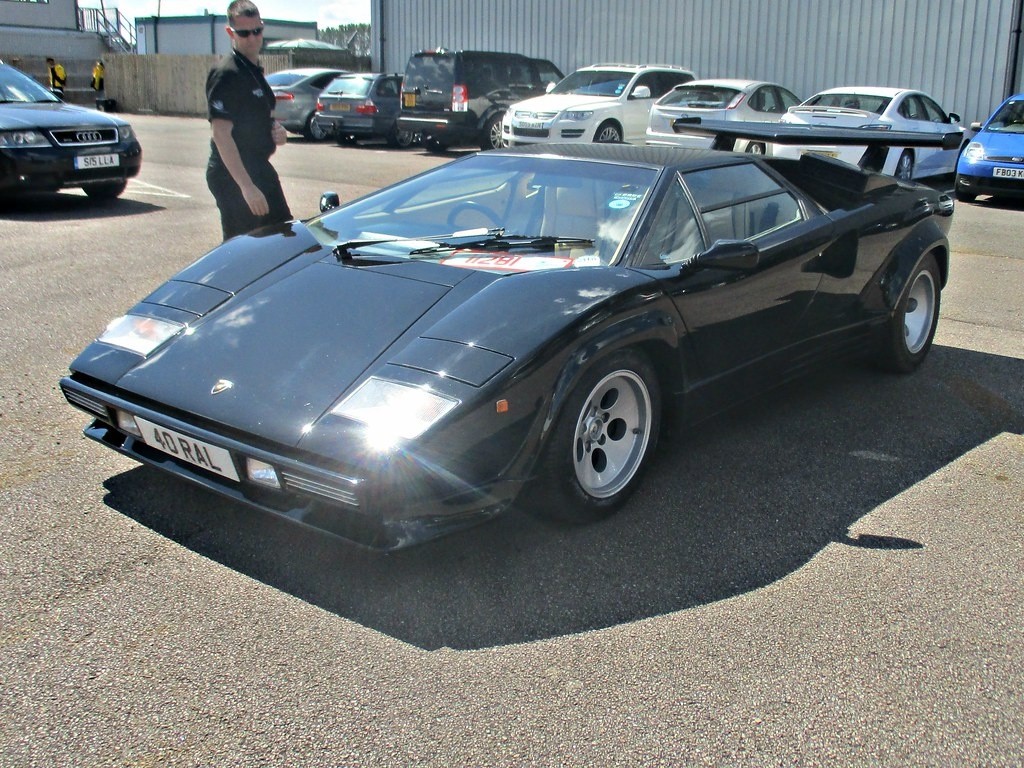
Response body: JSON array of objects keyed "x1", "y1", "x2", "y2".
[{"x1": 58, "y1": 142, "x2": 955, "y2": 554}]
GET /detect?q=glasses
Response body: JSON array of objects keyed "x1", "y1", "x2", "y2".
[{"x1": 231, "y1": 26, "x2": 262, "y2": 37}]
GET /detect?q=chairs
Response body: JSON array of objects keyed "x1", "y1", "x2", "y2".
[
  {"x1": 540, "y1": 178, "x2": 606, "y2": 256},
  {"x1": 651, "y1": 185, "x2": 751, "y2": 265}
]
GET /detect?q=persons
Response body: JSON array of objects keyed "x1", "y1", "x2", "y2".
[
  {"x1": 204, "y1": 1, "x2": 295, "y2": 244},
  {"x1": 90, "y1": 59, "x2": 105, "y2": 99},
  {"x1": 46, "y1": 58, "x2": 66, "y2": 99}
]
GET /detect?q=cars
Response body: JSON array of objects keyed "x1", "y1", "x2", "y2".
[
  {"x1": 264, "y1": 68, "x2": 355, "y2": 143},
  {"x1": 773, "y1": 87, "x2": 972, "y2": 181},
  {"x1": 1, "y1": 59, "x2": 142, "y2": 204},
  {"x1": 954, "y1": 92, "x2": 1024, "y2": 202},
  {"x1": 645, "y1": 79, "x2": 802, "y2": 155},
  {"x1": 315, "y1": 72, "x2": 422, "y2": 148}
]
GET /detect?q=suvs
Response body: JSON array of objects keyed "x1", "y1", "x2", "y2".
[
  {"x1": 502, "y1": 63, "x2": 701, "y2": 149},
  {"x1": 395, "y1": 49, "x2": 575, "y2": 151}
]
